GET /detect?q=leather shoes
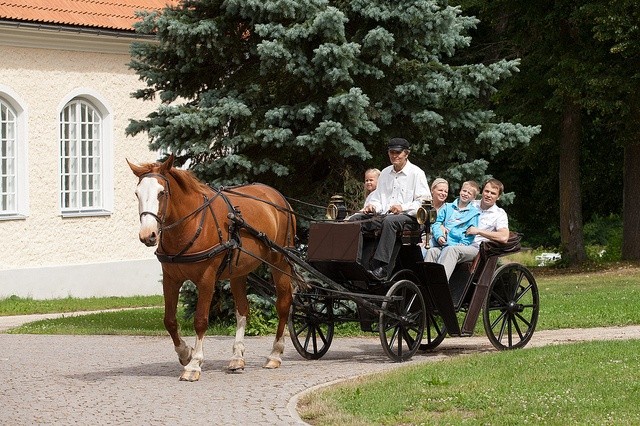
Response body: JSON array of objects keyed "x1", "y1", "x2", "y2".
[{"x1": 367, "y1": 266, "x2": 387, "y2": 282}]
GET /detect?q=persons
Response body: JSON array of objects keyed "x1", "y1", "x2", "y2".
[
  {"x1": 364, "y1": 168, "x2": 382, "y2": 200},
  {"x1": 437, "y1": 177, "x2": 510, "y2": 282},
  {"x1": 349, "y1": 136, "x2": 433, "y2": 285},
  {"x1": 425, "y1": 180, "x2": 480, "y2": 260},
  {"x1": 420, "y1": 177, "x2": 449, "y2": 257}
]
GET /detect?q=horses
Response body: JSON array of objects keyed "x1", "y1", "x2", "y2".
[{"x1": 123, "y1": 151, "x2": 300, "y2": 382}]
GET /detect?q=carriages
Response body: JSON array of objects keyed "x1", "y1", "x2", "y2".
[{"x1": 125, "y1": 153, "x2": 540, "y2": 382}]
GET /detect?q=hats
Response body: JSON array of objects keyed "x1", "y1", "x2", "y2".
[{"x1": 388, "y1": 137, "x2": 410, "y2": 151}]
